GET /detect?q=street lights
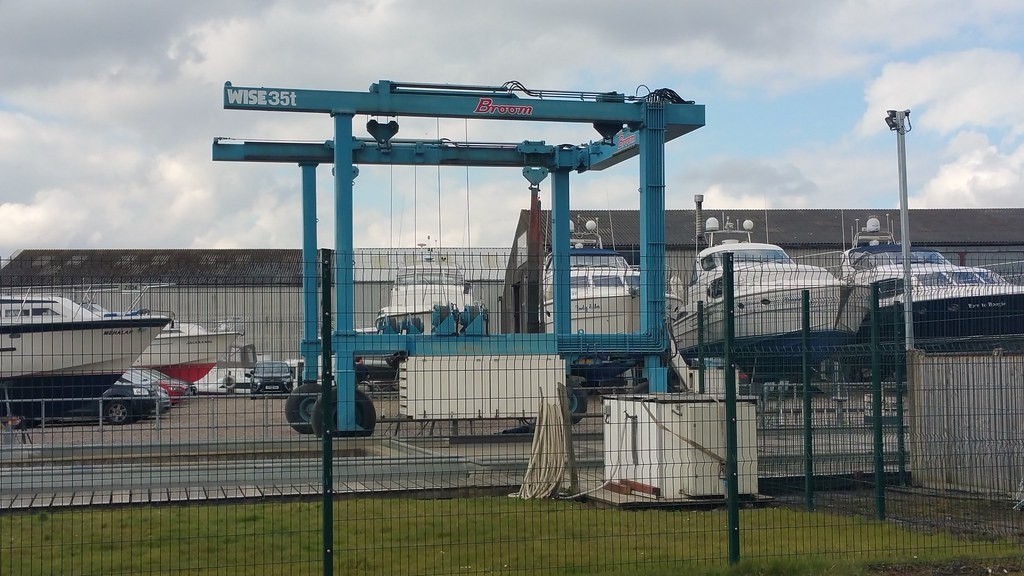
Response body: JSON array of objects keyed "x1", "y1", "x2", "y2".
[{"x1": 884, "y1": 108, "x2": 915, "y2": 355}]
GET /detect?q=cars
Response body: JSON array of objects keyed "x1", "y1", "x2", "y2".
[
  {"x1": 45, "y1": 371, "x2": 173, "y2": 425},
  {"x1": 129, "y1": 366, "x2": 198, "y2": 407}
]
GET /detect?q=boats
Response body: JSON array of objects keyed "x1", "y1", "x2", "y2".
[
  {"x1": 838, "y1": 207, "x2": 1024, "y2": 336},
  {"x1": 669, "y1": 194, "x2": 877, "y2": 351},
  {"x1": 0, "y1": 282, "x2": 175, "y2": 431},
  {"x1": 537, "y1": 210, "x2": 684, "y2": 340},
  {"x1": 73, "y1": 300, "x2": 239, "y2": 384},
  {"x1": 374, "y1": 236, "x2": 476, "y2": 335}
]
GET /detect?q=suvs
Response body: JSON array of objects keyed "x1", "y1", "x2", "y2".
[{"x1": 245, "y1": 361, "x2": 295, "y2": 400}]
